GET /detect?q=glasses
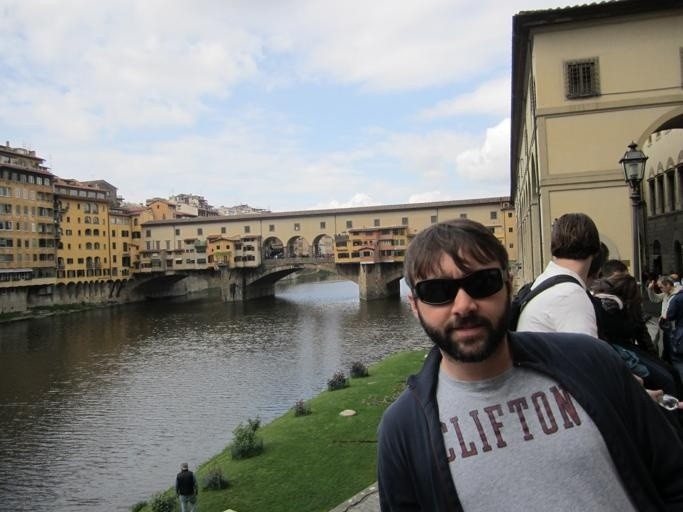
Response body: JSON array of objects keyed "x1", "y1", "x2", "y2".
[{"x1": 412, "y1": 266, "x2": 508, "y2": 305}]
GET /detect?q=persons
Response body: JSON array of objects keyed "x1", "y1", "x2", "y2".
[{"x1": 173, "y1": 462, "x2": 199, "y2": 512}]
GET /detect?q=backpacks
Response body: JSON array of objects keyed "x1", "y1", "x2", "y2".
[{"x1": 509, "y1": 275, "x2": 583, "y2": 331}]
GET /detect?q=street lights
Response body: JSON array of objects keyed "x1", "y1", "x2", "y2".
[{"x1": 618, "y1": 140, "x2": 649, "y2": 288}]
